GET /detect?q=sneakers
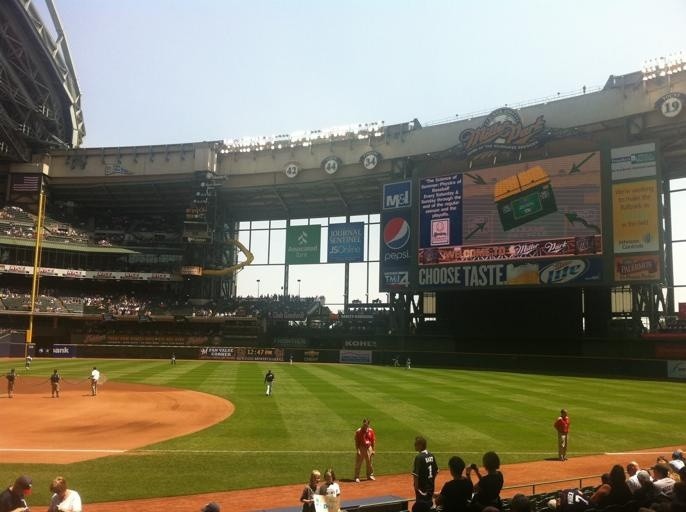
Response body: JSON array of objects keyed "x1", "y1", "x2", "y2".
[
  {"x1": 367, "y1": 476, "x2": 376, "y2": 481},
  {"x1": 266, "y1": 394, "x2": 271, "y2": 396},
  {"x1": 354, "y1": 475, "x2": 361, "y2": 483},
  {"x1": 561, "y1": 457, "x2": 568, "y2": 461},
  {"x1": 8, "y1": 393, "x2": 97, "y2": 399}
]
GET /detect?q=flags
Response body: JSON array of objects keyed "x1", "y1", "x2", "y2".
[{"x1": 12, "y1": 175, "x2": 38, "y2": 192}]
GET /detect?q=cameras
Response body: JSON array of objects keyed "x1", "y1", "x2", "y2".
[{"x1": 471, "y1": 464, "x2": 476, "y2": 469}]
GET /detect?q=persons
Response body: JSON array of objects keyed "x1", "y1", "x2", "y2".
[
  {"x1": 264, "y1": 370, "x2": 274, "y2": 396},
  {"x1": 25, "y1": 354, "x2": 32, "y2": 370},
  {"x1": 300, "y1": 418, "x2": 529, "y2": 512},
  {"x1": 88, "y1": 366, "x2": 99, "y2": 396},
  {"x1": 6, "y1": 367, "x2": 17, "y2": 398},
  {"x1": 289, "y1": 353, "x2": 293, "y2": 365},
  {"x1": 201, "y1": 500, "x2": 223, "y2": 512},
  {"x1": 554, "y1": 408, "x2": 570, "y2": 462},
  {"x1": 169, "y1": 351, "x2": 177, "y2": 365},
  {"x1": 51, "y1": 368, "x2": 61, "y2": 398},
  {"x1": 47, "y1": 476, "x2": 81, "y2": 512},
  {"x1": 548, "y1": 448, "x2": 686, "y2": 512},
  {"x1": 0, "y1": 475, "x2": 33, "y2": 512}
]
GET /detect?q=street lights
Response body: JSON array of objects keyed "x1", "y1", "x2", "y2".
[
  {"x1": 296, "y1": 279, "x2": 300, "y2": 299},
  {"x1": 256, "y1": 279, "x2": 260, "y2": 298}
]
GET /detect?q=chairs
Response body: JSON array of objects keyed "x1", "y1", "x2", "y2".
[{"x1": 432, "y1": 475, "x2": 685, "y2": 512}]
